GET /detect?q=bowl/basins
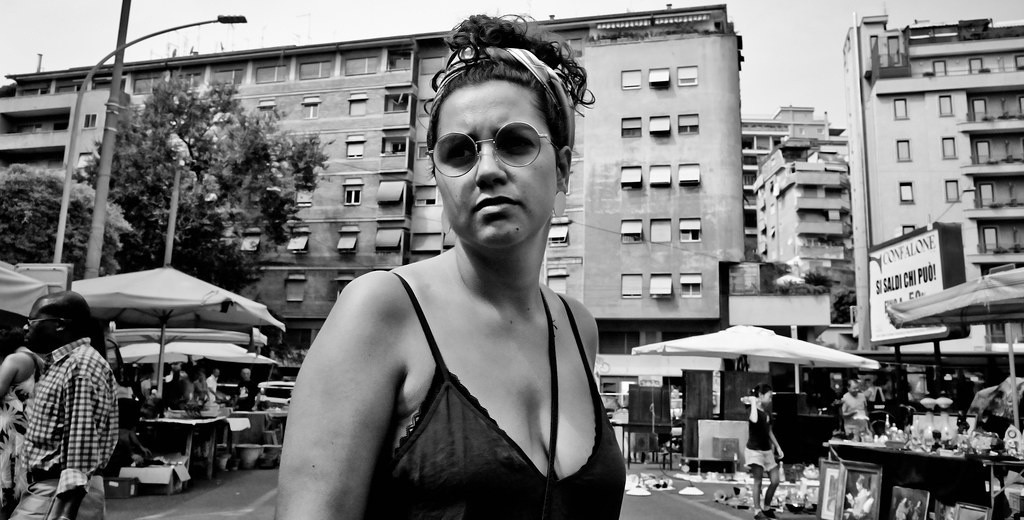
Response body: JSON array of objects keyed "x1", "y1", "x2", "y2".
[{"x1": 976, "y1": 446, "x2": 992, "y2": 455}]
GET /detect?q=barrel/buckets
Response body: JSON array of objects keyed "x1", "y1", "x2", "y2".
[{"x1": 215, "y1": 443, "x2": 282, "y2": 471}]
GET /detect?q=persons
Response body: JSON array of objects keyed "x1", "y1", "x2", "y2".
[
  {"x1": 846, "y1": 475, "x2": 874, "y2": 520},
  {"x1": 842, "y1": 380, "x2": 874, "y2": 443},
  {"x1": 0, "y1": 290, "x2": 265, "y2": 520},
  {"x1": 743, "y1": 384, "x2": 785, "y2": 519},
  {"x1": 861, "y1": 378, "x2": 885, "y2": 402},
  {"x1": 274, "y1": 15, "x2": 626, "y2": 520}
]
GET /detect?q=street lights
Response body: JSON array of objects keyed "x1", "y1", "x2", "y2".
[{"x1": 55, "y1": 14, "x2": 248, "y2": 259}]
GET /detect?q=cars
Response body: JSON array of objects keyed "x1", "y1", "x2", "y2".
[{"x1": 255, "y1": 380, "x2": 295, "y2": 412}]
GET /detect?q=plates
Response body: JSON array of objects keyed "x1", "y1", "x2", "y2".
[{"x1": 969, "y1": 451, "x2": 999, "y2": 456}]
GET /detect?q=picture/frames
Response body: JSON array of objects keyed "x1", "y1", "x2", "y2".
[
  {"x1": 835, "y1": 460, "x2": 882, "y2": 520},
  {"x1": 953, "y1": 502, "x2": 989, "y2": 520},
  {"x1": 816, "y1": 460, "x2": 840, "y2": 520}
]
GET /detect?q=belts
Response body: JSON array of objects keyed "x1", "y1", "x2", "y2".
[{"x1": 26, "y1": 463, "x2": 102, "y2": 485}]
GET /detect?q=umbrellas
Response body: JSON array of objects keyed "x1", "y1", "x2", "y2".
[
  {"x1": 71, "y1": 263, "x2": 286, "y2": 399},
  {"x1": 631, "y1": 323, "x2": 881, "y2": 401},
  {"x1": 106, "y1": 340, "x2": 279, "y2": 378},
  {"x1": 886, "y1": 266, "x2": 1024, "y2": 329}
]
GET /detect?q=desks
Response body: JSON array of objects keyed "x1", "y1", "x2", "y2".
[
  {"x1": 680, "y1": 456, "x2": 740, "y2": 479},
  {"x1": 612, "y1": 422, "x2": 685, "y2": 470},
  {"x1": 142, "y1": 416, "x2": 226, "y2": 487},
  {"x1": 227, "y1": 411, "x2": 288, "y2": 470},
  {"x1": 824, "y1": 442, "x2": 1024, "y2": 520}
]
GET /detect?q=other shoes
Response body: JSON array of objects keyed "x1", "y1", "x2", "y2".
[{"x1": 754, "y1": 508, "x2": 776, "y2": 520}]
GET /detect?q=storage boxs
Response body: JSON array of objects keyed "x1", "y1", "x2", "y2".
[
  {"x1": 120, "y1": 454, "x2": 192, "y2": 496},
  {"x1": 105, "y1": 477, "x2": 139, "y2": 500}
]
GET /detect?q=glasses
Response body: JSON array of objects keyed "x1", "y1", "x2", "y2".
[
  {"x1": 425, "y1": 122, "x2": 561, "y2": 178},
  {"x1": 24, "y1": 330, "x2": 29, "y2": 333},
  {"x1": 27, "y1": 318, "x2": 59, "y2": 326}
]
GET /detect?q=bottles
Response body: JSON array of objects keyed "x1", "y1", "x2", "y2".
[{"x1": 778, "y1": 461, "x2": 786, "y2": 483}]
